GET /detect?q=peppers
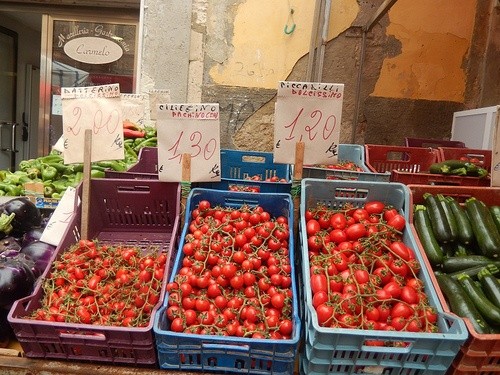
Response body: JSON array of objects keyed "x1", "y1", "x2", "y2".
[{"x1": 0, "y1": 119, "x2": 157, "y2": 199}]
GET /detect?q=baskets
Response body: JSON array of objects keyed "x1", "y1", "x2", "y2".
[
  {"x1": 105, "y1": 145, "x2": 158, "y2": 180},
  {"x1": 154, "y1": 187, "x2": 303, "y2": 375},
  {"x1": 301, "y1": 178, "x2": 468, "y2": 375},
  {"x1": 408, "y1": 183, "x2": 500, "y2": 375},
  {"x1": 7, "y1": 179, "x2": 183, "y2": 363},
  {"x1": 301, "y1": 144, "x2": 378, "y2": 181},
  {"x1": 404, "y1": 137, "x2": 465, "y2": 148},
  {"x1": 365, "y1": 145, "x2": 492, "y2": 187},
  {"x1": 196, "y1": 149, "x2": 293, "y2": 193}
]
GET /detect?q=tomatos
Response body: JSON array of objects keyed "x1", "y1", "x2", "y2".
[
  {"x1": 166, "y1": 200, "x2": 292, "y2": 369},
  {"x1": 229, "y1": 175, "x2": 288, "y2": 192},
  {"x1": 306, "y1": 201, "x2": 440, "y2": 362},
  {"x1": 19, "y1": 240, "x2": 167, "y2": 327},
  {"x1": 303, "y1": 161, "x2": 367, "y2": 198}
]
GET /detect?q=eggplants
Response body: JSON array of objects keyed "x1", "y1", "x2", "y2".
[
  {"x1": 415, "y1": 192, "x2": 500, "y2": 334},
  {"x1": 429, "y1": 160, "x2": 488, "y2": 176},
  {"x1": 0, "y1": 198, "x2": 56, "y2": 345}
]
